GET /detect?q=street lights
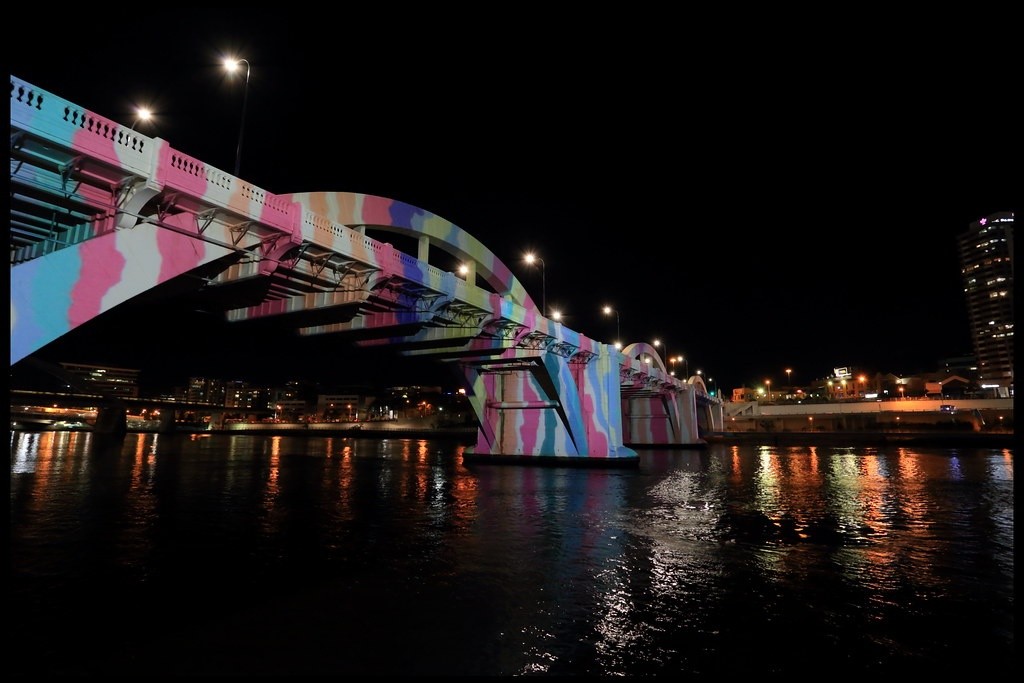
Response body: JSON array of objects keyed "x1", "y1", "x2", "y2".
[
  {"x1": 671, "y1": 357, "x2": 675, "y2": 374},
  {"x1": 859, "y1": 376, "x2": 867, "y2": 399},
  {"x1": 786, "y1": 369, "x2": 791, "y2": 394},
  {"x1": 678, "y1": 357, "x2": 688, "y2": 383},
  {"x1": 697, "y1": 371, "x2": 706, "y2": 386},
  {"x1": 709, "y1": 378, "x2": 716, "y2": 397},
  {"x1": 766, "y1": 380, "x2": 770, "y2": 403},
  {"x1": 654, "y1": 340, "x2": 667, "y2": 371},
  {"x1": 524, "y1": 253, "x2": 545, "y2": 316},
  {"x1": 604, "y1": 307, "x2": 620, "y2": 348}
]
